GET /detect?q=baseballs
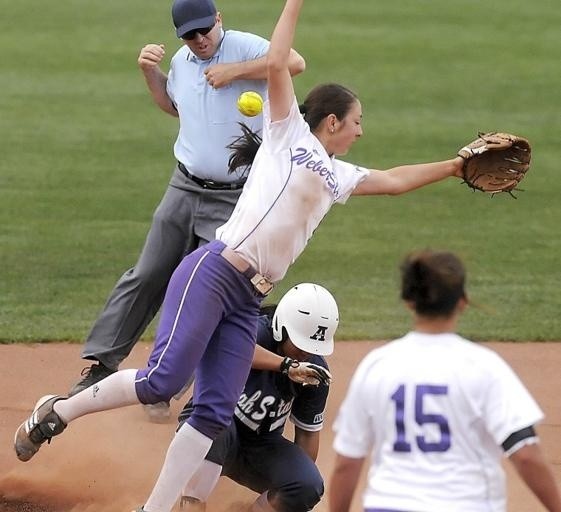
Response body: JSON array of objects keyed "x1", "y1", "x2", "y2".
[{"x1": 238, "y1": 91, "x2": 263, "y2": 116}]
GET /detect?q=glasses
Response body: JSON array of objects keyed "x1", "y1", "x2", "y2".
[{"x1": 181, "y1": 22, "x2": 214, "y2": 40}]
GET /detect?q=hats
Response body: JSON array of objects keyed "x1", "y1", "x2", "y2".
[{"x1": 172, "y1": 0, "x2": 216, "y2": 38}]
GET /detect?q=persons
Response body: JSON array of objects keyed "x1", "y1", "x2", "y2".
[
  {"x1": 327, "y1": 246, "x2": 560, "y2": 512},
  {"x1": 176, "y1": 281, "x2": 341, "y2": 512},
  {"x1": 67, "y1": 1, "x2": 307, "y2": 424},
  {"x1": 13, "y1": 2, "x2": 533, "y2": 512}
]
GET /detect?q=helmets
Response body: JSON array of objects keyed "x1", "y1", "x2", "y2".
[{"x1": 271, "y1": 283, "x2": 339, "y2": 356}]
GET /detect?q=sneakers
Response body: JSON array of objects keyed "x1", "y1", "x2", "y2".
[
  {"x1": 14, "y1": 395, "x2": 68, "y2": 461},
  {"x1": 68, "y1": 364, "x2": 117, "y2": 397}
]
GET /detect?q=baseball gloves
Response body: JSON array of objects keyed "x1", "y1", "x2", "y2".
[{"x1": 459, "y1": 133, "x2": 531, "y2": 192}]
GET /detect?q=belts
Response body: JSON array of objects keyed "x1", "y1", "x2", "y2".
[
  {"x1": 220, "y1": 246, "x2": 274, "y2": 296},
  {"x1": 178, "y1": 160, "x2": 247, "y2": 190}
]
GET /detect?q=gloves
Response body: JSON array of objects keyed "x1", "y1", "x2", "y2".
[{"x1": 279, "y1": 356, "x2": 334, "y2": 387}]
